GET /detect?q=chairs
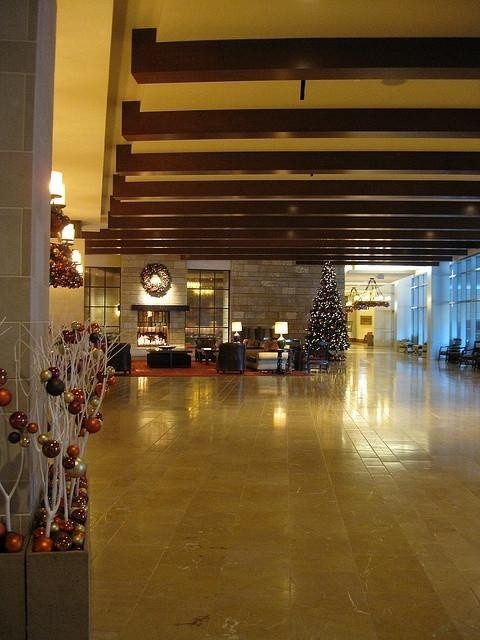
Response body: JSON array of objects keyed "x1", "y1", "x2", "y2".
[
  {"x1": 107, "y1": 343, "x2": 131, "y2": 376},
  {"x1": 399, "y1": 335, "x2": 427, "y2": 359},
  {"x1": 195, "y1": 337, "x2": 216, "y2": 365},
  {"x1": 217, "y1": 342, "x2": 246, "y2": 375},
  {"x1": 438, "y1": 338, "x2": 480, "y2": 372},
  {"x1": 307, "y1": 342, "x2": 330, "y2": 374}
]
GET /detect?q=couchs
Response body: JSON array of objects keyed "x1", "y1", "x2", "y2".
[{"x1": 246, "y1": 348, "x2": 278, "y2": 374}]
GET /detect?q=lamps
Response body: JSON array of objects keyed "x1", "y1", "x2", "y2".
[
  {"x1": 48, "y1": 171, "x2": 67, "y2": 215},
  {"x1": 274, "y1": 322, "x2": 289, "y2": 349},
  {"x1": 345, "y1": 278, "x2": 390, "y2": 313},
  {"x1": 71, "y1": 249, "x2": 82, "y2": 268},
  {"x1": 62, "y1": 222, "x2": 75, "y2": 250},
  {"x1": 232, "y1": 321, "x2": 242, "y2": 337}
]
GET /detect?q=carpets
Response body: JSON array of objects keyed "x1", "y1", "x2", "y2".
[{"x1": 116, "y1": 357, "x2": 315, "y2": 376}]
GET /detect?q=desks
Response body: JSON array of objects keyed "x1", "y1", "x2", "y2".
[{"x1": 146, "y1": 350, "x2": 192, "y2": 369}]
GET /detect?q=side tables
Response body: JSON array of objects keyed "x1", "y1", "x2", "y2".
[{"x1": 276, "y1": 349, "x2": 293, "y2": 374}]
